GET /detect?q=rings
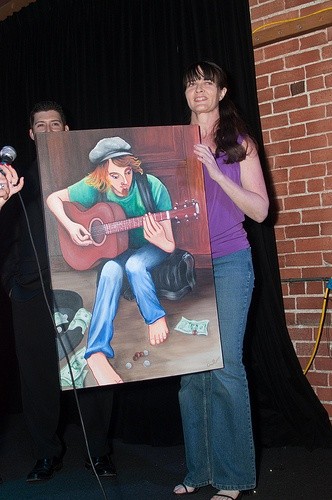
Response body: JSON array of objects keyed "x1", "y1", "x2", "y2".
[{"x1": 0, "y1": 184, "x2": 4, "y2": 189}]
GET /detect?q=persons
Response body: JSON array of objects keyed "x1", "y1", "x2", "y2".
[
  {"x1": 0, "y1": 101, "x2": 118, "y2": 482},
  {"x1": 172, "y1": 59, "x2": 271, "y2": 500}
]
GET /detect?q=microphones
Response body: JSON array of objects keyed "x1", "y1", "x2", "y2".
[{"x1": 0, "y1": 146, "x2": 17, "y2": 189}]
